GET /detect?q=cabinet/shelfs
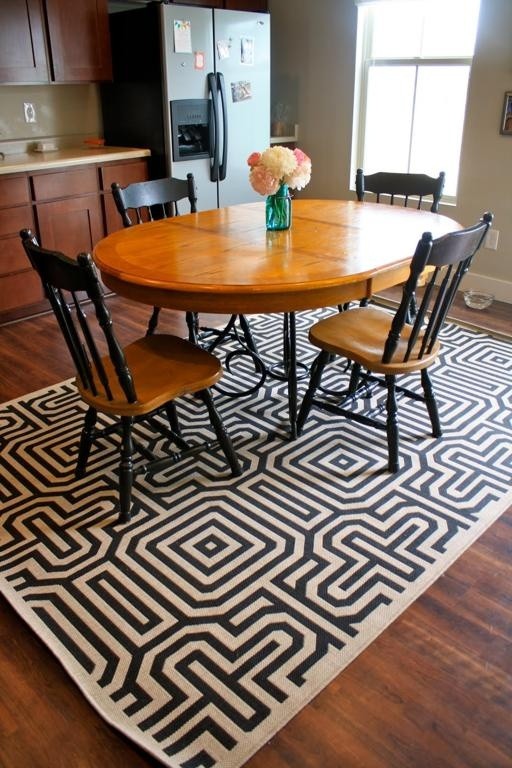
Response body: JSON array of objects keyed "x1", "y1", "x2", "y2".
[
  {"x1": 0, "y1": 0, "x2": 114, "y2": 87},
  {"x1": 271, "y1": 141, "x2": 295, "y2": 199},
  {"x1": 0, "y1": 157, "x2": 149, "y2": 315}
]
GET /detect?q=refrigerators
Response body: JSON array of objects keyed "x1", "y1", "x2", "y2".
[{"x1": 98, "y1": 4, "x2": 271, "y2": 221}]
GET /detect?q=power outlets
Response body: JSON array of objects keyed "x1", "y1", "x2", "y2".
[{"x1": 24, "y1": 102, "x2": 36, "y2": 124}]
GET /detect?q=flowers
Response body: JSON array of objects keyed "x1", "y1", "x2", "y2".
[{"x1": 248, "y1": 145, "x2": 313, "y2": 197}]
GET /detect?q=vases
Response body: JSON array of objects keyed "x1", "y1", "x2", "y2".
[{"x1": 265, "y1": 184, "x2": 292, "y2": 230}]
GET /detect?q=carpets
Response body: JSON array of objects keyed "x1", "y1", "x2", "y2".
[{"x1": 0, "y1": 290, "x2": 512, "y2": 767}]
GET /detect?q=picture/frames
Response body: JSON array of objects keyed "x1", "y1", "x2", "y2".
[{"x1": 499, "y1": 91, "x2": 512, "y2": 135}]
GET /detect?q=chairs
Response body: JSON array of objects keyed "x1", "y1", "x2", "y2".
[
  {"x1": 292, "y1": 212, "x2": 493, "y2": 473},
  {"x1": 329, "y1": 168, "x2": 444, "y2": 360},
  {"x1": 21, "y1": 230, "x2": 244, "y2": 523},
  {"x1": 111, "y1": 172, "x2": 263, "y2": 360}
]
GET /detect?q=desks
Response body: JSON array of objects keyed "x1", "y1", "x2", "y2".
[{"x1": 90, "y1": 199, "x2": 463, "y2": 439}]
